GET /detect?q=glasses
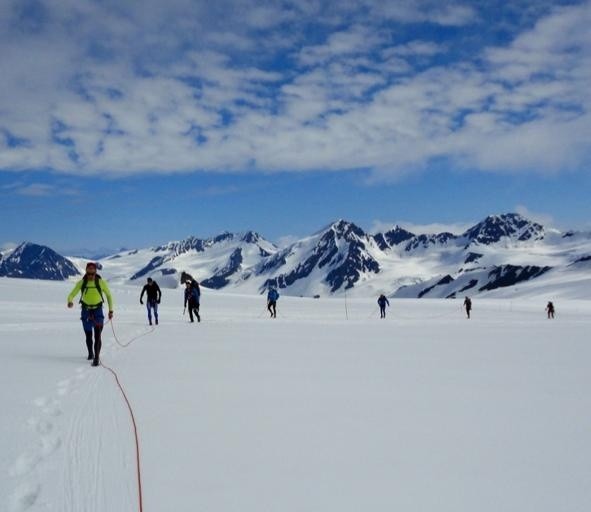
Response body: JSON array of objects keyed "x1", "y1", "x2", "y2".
[{"x1": 86, "y1": 273, "x2": 95, "y2": 275}]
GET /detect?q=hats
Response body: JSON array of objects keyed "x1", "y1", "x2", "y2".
[{"x1": 86, "y1": 262, "x2": 96, "y2": 273}]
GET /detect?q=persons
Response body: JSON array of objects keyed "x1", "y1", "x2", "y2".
[
  {"x1": 183, "y1": 279, "x2": 199, "y2": 323},
  {"x1": 138, "y1": 277, "x2": 160, "y2": 326},
  {"x1": 462, "y1": 296, "x2": 471, "y2": 319},
  {"x1": 266, "y1": 286, "x2": 278, "y2": 318},
  {"x1": 377, "y1": 294, "x2": 389, "y2": 319},
  {"x1": 67, "y1": 262, "x2": 114, "y2": 366},
  {"x1": 545, "y1": 301, "x2": 555, "y2": 319}
]
